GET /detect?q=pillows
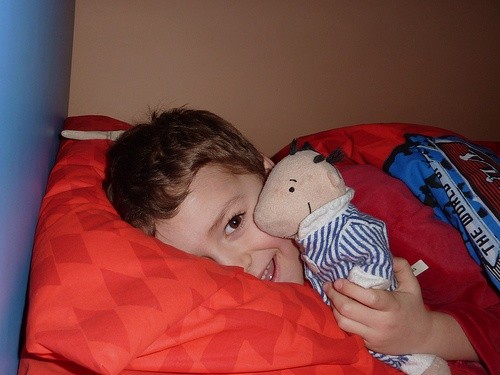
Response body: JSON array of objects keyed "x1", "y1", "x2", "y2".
[{"x1": 26, "y1": 116, "x2": 357, "y2": 375}]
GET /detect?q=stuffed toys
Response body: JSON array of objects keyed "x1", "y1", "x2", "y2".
[{"x1": 253, "y1": 137, "x2": 452, "y2": 375}]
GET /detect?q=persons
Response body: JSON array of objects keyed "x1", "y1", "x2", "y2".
[{"x1": 103, "y1": 102, "x2": 500, "y2": 375}]
